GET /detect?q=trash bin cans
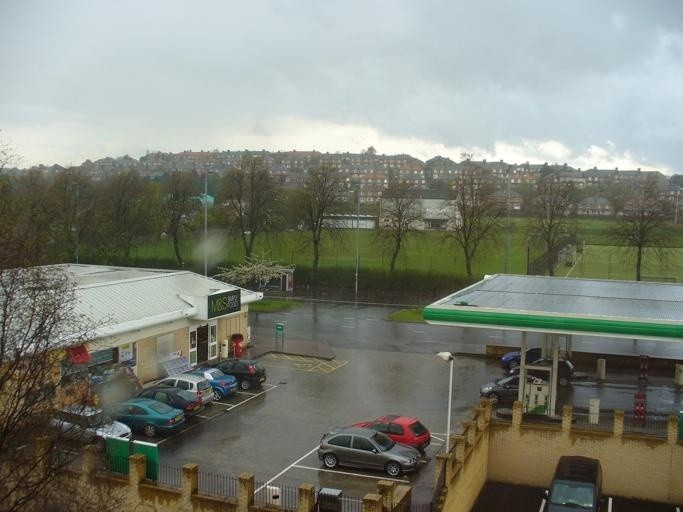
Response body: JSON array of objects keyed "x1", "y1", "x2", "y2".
[
  {"x1": 231, "y1": 334, "x2": 243, "y2": 357},
  {"x1": 221, "y1": 339, "x2": 229, "y2": 359}
]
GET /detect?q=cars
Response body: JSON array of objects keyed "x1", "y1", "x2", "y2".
[
  {"x1": 478, "y1": 344, "x2": 578, "y2": 404},
  {"x1": 352, "y1": 414, "x2": 431, "y2": 451},
  {"x1": 317, "y1": 426, "x2": 422, "y2": 478},
  {"x1": 114, "y1": 356, "x2": 266, "y2": 438}
]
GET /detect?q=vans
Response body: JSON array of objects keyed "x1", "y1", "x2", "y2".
[{"x1": 49, "y1": 405, "x2": 133, "y2": 454}]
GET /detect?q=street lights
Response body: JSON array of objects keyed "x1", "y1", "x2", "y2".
[{"x1": 436, "y1": 350, "x2": 454, "y2": 453}]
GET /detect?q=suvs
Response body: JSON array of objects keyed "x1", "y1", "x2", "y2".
[{"x1": 541, "y1": 454, "x2": 606, "y2": 512}]
